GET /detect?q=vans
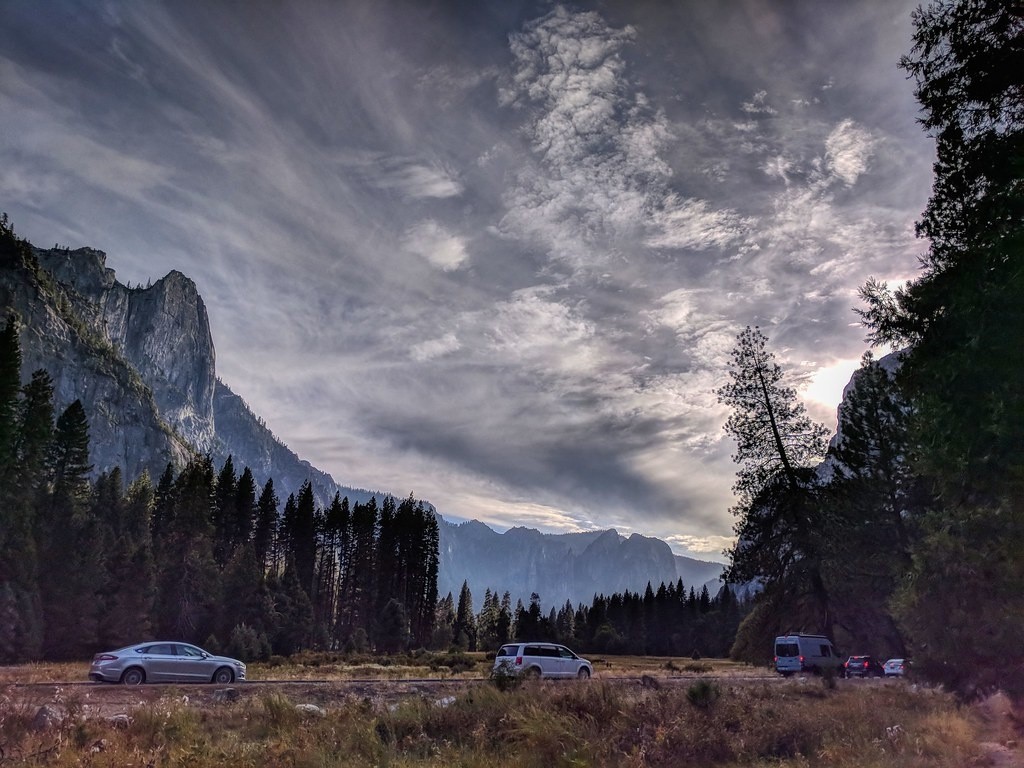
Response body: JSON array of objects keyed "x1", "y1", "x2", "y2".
[{"x1": 774, "y1": 632, "x2": 843, "y2": 678}]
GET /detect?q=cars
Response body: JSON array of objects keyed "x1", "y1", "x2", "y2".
[
  {"x1": 492, "y1": 641, "x2": 594, "y2": 681},
  {"x1": 883, "y1": 658, "x2": 912, "y2": 678},
  {"x1": 842, "y1": 655, "x2": 885, "y2": 679},
  {"x1": 88, "y1": 640, "x2": 247, "y2": 686}
]
